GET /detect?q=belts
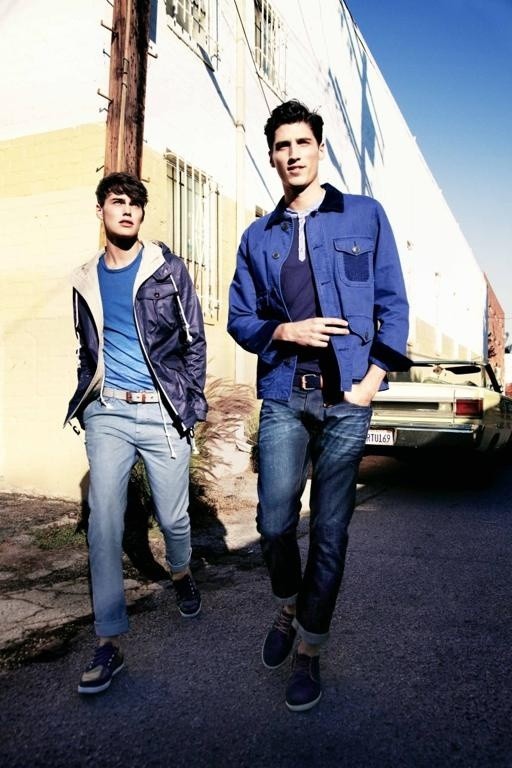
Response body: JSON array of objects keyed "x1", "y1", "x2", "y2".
[
  {"x1": 293, "y1": 373, "x2": 324, "y2": 392},
  {"x1": 102, "y1": 388, "x2": 159, "y2": 403}
]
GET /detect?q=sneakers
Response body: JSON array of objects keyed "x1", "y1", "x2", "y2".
[
  {"x1": 262, "y1": 604, "x2": 300, "y2": 670},
  {"x1": 285, "y1": 640, "x2": 322, "y2": 712},
  {"x1": 78, "y1": 641, "x2": 124, "y2": 694},
  {"x1": 169, "y1": 566, "x2": 201, "y2": 618}
]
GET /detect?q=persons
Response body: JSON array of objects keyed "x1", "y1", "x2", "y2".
[
  {"x1": 224, "y1": 100, "x2": 410, "y2": 716},
  {"x1": 69, "y1": 173, "x2": 208, "y2": 698}
]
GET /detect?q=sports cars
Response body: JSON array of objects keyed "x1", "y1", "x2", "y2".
[{"x1": 351, "y1": 361, "x2": 512, "y2": 466}]
